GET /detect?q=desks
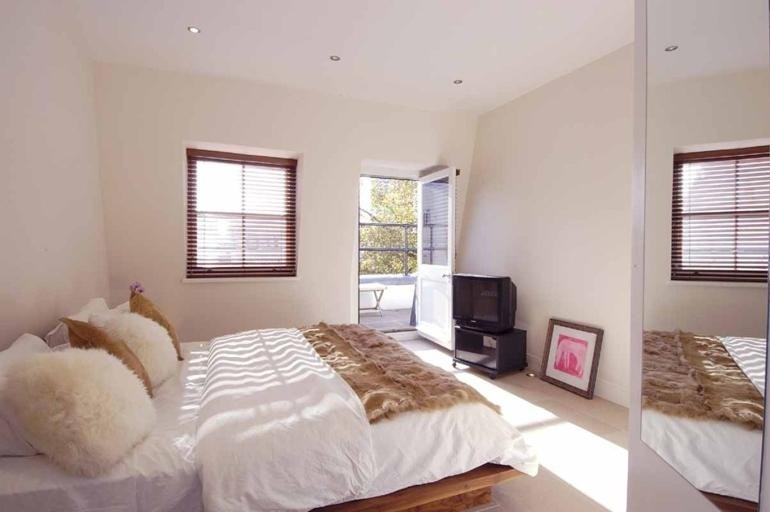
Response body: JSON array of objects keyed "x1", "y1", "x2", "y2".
[{"x1": 360, "y1": 283, "x2": 386, "y2": 318}]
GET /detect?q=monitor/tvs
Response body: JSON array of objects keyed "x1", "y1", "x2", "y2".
[{"x1": 453, "y1": 273, "x2": 516, "y2": 333}]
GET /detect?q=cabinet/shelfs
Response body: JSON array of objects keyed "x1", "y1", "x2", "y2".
[{"x1": 452, "y1": 327, "x2": 527, "y2": 380}]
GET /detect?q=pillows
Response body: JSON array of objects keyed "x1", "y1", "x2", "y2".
[{"x1": 0, "y1": 282, "x2": 182, "y2": 479}]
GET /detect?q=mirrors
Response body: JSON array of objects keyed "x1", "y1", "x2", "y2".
[{"x1": 639, "y1": 1, "x2": 770, "y2": 512}]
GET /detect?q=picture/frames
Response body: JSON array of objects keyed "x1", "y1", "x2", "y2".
[{"x1": 538, "y1": 317, "x2": 605, "y2": 400}]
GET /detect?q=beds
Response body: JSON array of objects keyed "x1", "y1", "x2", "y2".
[
  {"x1": 0, "y1": 323, "x2": 496, "y2": 512},
  {"x1": 641, "y1": 331, "x2": 770, "y2": 503}
]
